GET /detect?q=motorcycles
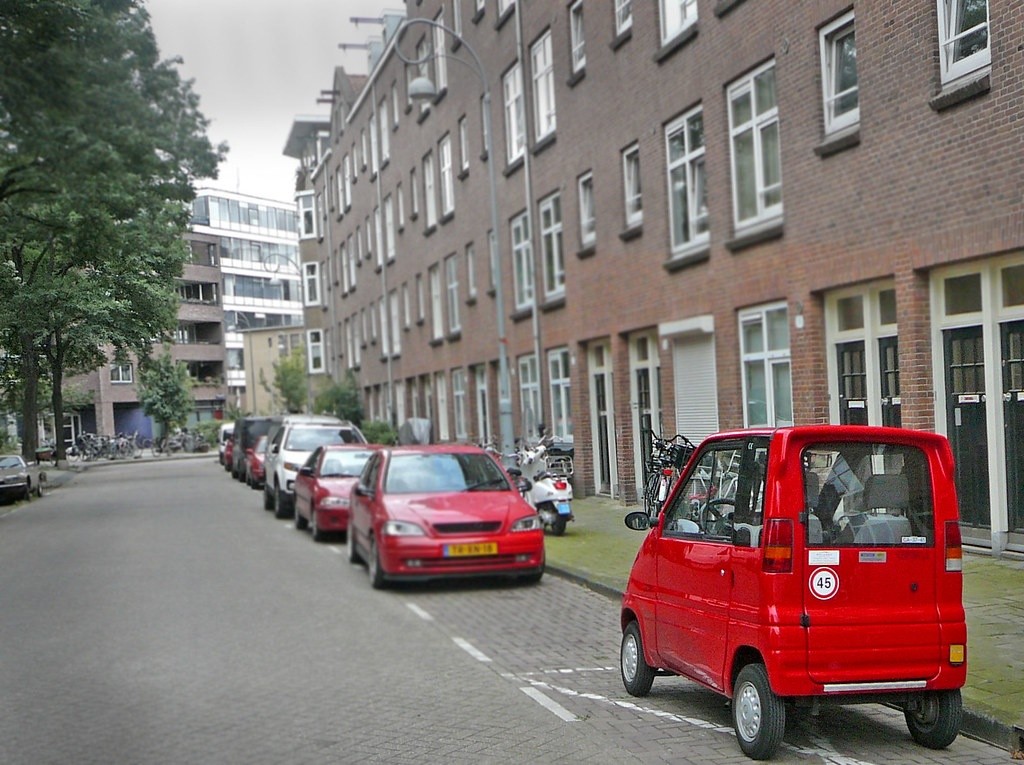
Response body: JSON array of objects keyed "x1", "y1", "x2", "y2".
[{"x1": 512, "y1": 427, "x2": 576, "y2": 538}]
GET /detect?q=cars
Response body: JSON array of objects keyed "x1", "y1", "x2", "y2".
[
  {"x1": 0, "y1": 453, "x2": 47, "y2": 503},
  {"x1": 217, "y1": 416, "x2": 275, "y2": 488},
  {"x1": 293, "y1": 443, "x2": 391, "y2": 542},
  {"x1": 345, "y1": 443, "x2": 547, "y2": 590},
  {"x1": 617, "y1": 425, "x2": 969, "y2": 760}
]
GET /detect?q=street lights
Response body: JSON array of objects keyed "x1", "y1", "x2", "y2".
[{"x1": 393, "y1": 19, "x2": 519, "y2": 465}]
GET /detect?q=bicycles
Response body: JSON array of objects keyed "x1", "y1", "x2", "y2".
[
  {"x1": 37, "y1": 427, "x2": 207, "y2": 465},
  {"x1": 637, "y1": 423, "x2": 779, "y2": 544}
]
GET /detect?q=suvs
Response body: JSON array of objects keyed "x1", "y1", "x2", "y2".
[{"x1": 261, "y1": 416, "x2": 367, "y2": 521}]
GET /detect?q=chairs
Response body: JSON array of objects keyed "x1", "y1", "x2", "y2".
[
  {"x1": 803, "y1": 471, "x2": 824, "y2": 544},
  {"x1": 833, "y1": 473, "x2": 912, "y2": 543}
]
